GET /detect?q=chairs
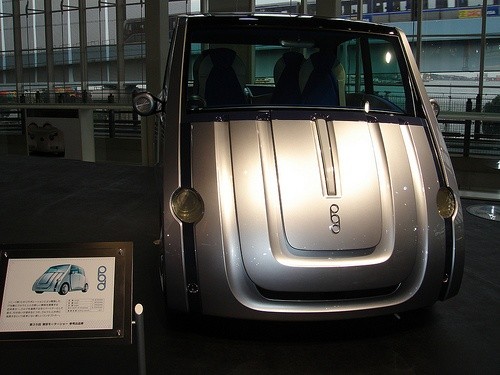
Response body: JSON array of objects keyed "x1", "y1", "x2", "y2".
[
  {"x1": 274, "y1": 54, "x2": 308, "y2": 109},
  {"x1": 193, "y1": 48, "x2": 254, "y2": 108},
  {"x1": 298, "y1": 51, "x2": 349, "y2": 109}
]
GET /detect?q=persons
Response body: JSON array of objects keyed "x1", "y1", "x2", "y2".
[{"x1": 31, "y1": 86, "x2": 91, "y2": 106}]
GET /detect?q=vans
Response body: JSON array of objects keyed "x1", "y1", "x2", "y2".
[{"x1": 133, "y1": 10, "x2": 467, "y2": 321}]
